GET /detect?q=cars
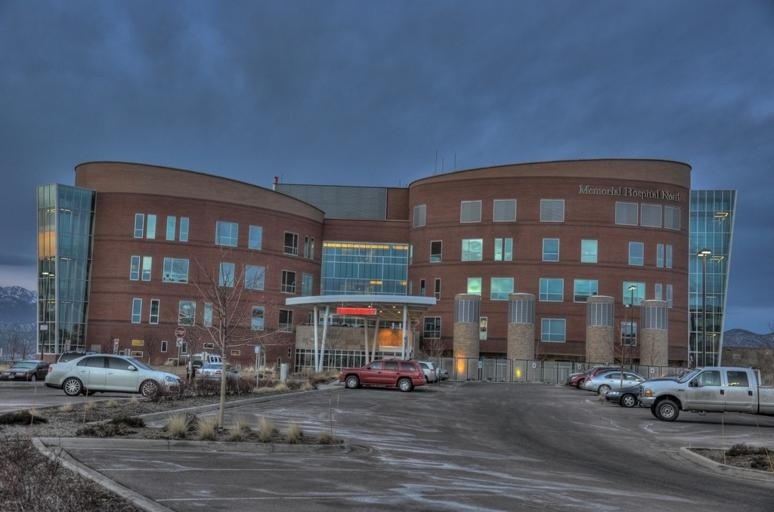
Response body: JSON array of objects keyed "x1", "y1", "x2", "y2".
[
  {"x1": 44, "y1": 350, "x2": 183, "y2": 398},
  {"x1": 195, "y1": 363, "x2": 241, "y2": 381},
  {"x1": 1, "y1": 360, "x2": 49, "y2": 382},
  {"x1": 569, "y1": 364, "x2": 680, "y2": 407}
]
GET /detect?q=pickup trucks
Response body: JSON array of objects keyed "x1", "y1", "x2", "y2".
[{"x1": 638, "y1": 365, "x2": 774, "y2": 421}]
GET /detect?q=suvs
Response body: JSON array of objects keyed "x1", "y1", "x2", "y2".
[{"x1": 338, "y1": 357, "x2": 448, "y2": 392}]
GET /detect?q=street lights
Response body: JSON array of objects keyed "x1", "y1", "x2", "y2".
[
  {"x1": 696, "y1": 249, "x2": 711, "y2": 365},
  {"x1": 627, "y1": 285, "x2": 636, "y2": 369}
]
